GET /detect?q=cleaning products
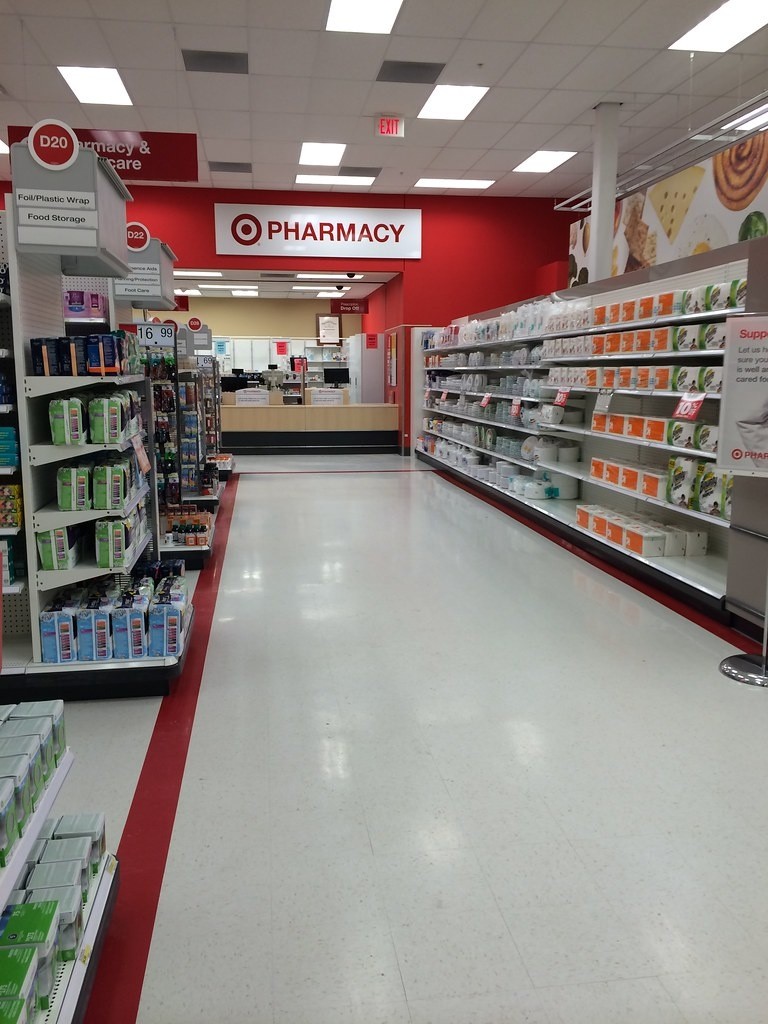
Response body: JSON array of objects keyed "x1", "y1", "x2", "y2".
[{"x1": 416, "y1": 432, "x2": 465, "y2": 468}]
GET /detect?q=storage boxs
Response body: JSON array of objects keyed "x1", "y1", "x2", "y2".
[
  {"x1": 607, "y1": 517, "x2": 638, "y2": 546},
  {"x1": 589, "y1": 456, "x2": 669, "y2": 499},
  {"x1": 591, "y1": 510, "x2": 623, "y2": 538},
  {"x1": 694, "y1": 461, "x2": 724, "y2": 518},
  {"x1": 625, "y1": 524, "x2": 666, "y2": 556},
  {"x1": 654, "y1": 516, "x2": 683, "y2": 524},
  {"x1": 634, "y1": 509, "x2": 660, "y2": 517},
  {"x1": 621, "y1": 510, "x2": 646, "y2": 520},
  {"x1": 599, "y1": 502, "x2": 623, "y2": 512},
  {"x1": 637, "y1": 517, "x2": 664, "y2": 526},
  {"x1": 651, "y1": 525, "x2": 687, "y2": 557},
  {"x1": 720, "y1": 473, "x2": 735, "y2": 520},
  {"x1": 0, "y1": 259, "x2": 234, "y2": 663},
  {"x1": 574, "y1": 504, "x2": 605, "y2": 529},
  {"x1": 668, "y1": 455, "x2": 709, "y2": 511},
  {"x1": 540, "y1": 277, "x2": 747, "y2": 452},
  {"x1": 667, "y1": 524, "x2": 708, "y2": 556}
]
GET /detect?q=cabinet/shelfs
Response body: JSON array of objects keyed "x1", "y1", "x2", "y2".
[
  {"x1": 304, "y1": 346, "x2": 349, "y2": 382},
  {"x1": 179, "y1": 371, "x2": 225, "y2": 515},
  {"x1": 177, "y1": 328, "x2": 234, "y2": 484},
  {"x1": 355, "y1": 333, "x2": 384, "y2": 404},
  {"x1": 414, "y1": 236, "x2": 768, "y2": 644},
  {"x1": 0, "y1": 142, "x2": 196, "y2": 701},
  {"x1": 348, "y1": 335, "x2": 355, "y2": 404},
  {"x1": 62, "y1": 238, "x2": 215, "y2": 570}
]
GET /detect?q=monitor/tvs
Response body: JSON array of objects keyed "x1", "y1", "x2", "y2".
[
  {"x1": 324, "y1": 368, "x2": 349, "y2": 389},
  {"x1": 262, "y1": 370, "x2": 283, "y2": 386},
  {"x1": 221, "y1": 376, "x2": 248, "y2": 393}
]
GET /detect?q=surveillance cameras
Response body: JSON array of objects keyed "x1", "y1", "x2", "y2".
[
  {"x1": 336, "y1": 286, "x2": 343, "y2": 290},
  {"x1": 347, "y1": 273, "x2": 355, "y2": 278}
]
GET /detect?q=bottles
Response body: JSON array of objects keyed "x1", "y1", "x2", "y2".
[
  {"x1": 165, "y1": 524, "x2": 208, "y2": 546},
  {"x1": 139, "y1": 352, "x2": 176, "y2": 381},
  {"x1": 153, "y1": 384, "x2": 181, "y2": 506}
]
